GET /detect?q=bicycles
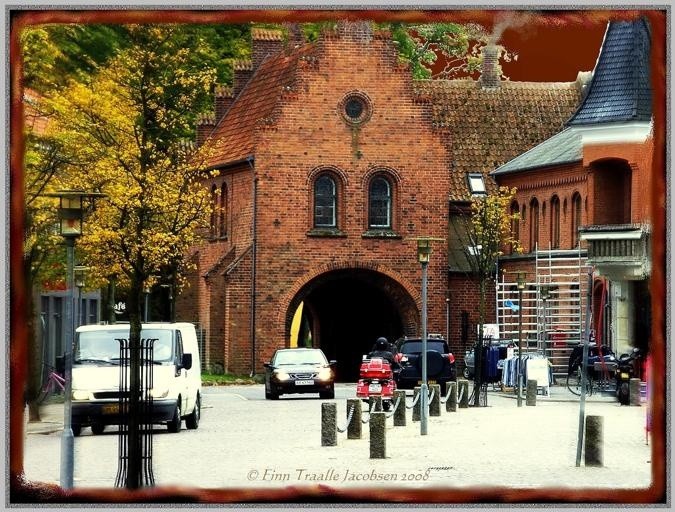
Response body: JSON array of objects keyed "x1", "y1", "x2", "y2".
[{"x1": 564, "y1": 355, "x2": 596, "y2": 399}]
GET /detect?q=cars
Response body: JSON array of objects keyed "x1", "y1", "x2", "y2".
[{"x1": 262, "y1": 346, "x2": 339, "y2": 400}]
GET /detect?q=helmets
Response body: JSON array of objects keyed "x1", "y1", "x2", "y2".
[{"x1": 376, "y1": 337, "x2": 388, "y2": 350}]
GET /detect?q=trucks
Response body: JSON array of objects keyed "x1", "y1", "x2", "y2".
[{"x1": 70, "y1": 314, "x2": 207, "y2": 436}]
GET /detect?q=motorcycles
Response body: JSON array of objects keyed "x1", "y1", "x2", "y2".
[{"x1": 354, "y1": 354, "x2": 413, "y2": 412}]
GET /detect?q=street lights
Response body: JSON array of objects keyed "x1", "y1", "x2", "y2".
[
  {"x1": 403, "y1": 233, "x2": 448, "y2": 438},
  {"x1": 506, "y1": 268, "x2": 538, "y2": 409},
  {"x1": 25, "y1": 184, "x2": 117, "y2": 496}
]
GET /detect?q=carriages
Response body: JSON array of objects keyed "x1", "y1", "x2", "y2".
[{"x1": 608, "y1": 345, "x2": 643, "y2": 406}]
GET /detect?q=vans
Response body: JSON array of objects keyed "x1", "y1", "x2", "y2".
[{"x1": 461, "y1": 338, "x2": 519, "y2": 381}]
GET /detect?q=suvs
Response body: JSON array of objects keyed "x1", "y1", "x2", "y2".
[{"x1": 390, "y1": 332, "x2": 461, "y2": 394}]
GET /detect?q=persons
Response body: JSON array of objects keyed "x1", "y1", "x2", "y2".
[{"x1": 363, "y1": 336, "x2": 406, "y2": 386}]
